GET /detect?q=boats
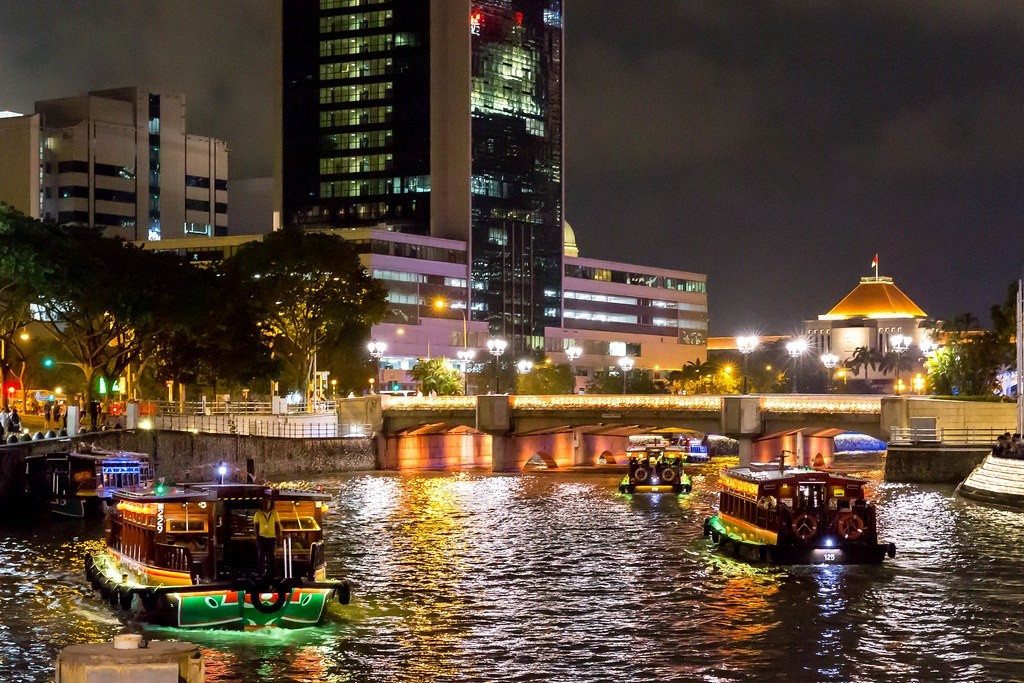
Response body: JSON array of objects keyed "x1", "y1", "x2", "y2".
[
  {"x1": 83, "y1": 457, "x2": 361, "y2": 633},
  {"x1": 673, "y1": 435, "x2": 712, "y2": 464},
  {"x1": 619, "y1": 434, "x2": 693, "y2": 496},
  {"x1": 20, "y1": 442, "x2": 156, "y2": 522},
  {"x1": 702, "y1": 450, "x2": 896, "y2": 567}
]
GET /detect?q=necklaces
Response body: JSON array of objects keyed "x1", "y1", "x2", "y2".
[{"x1": 261, "y1": 509, "x2": 272, "y2": 529}]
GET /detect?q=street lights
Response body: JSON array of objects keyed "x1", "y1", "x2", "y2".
[
  {"x1": 488, "y1": 339, "x2": 508, "y2": 394},
  {"x1": 891, "y1": 334, "x2": 912, "y2": 395},
  {"x1": 331, "y1": 379, "x2": 336, "y2": 400},
  {"x1": 435, "y1": 300, "x2": 470, "y2": 396},
  {"x1": 820, "y1": 352, "x2": 840, "y2": 393},
  {"x1": 786, "y1": 339, "x2": 808, "y2": 394},
  {"x1": 368, "y1": 378, "x2": 375, "y2": 394},
  {"x1": 618, "y1": 357, "x2": 635, "y2": 394},
  {"x1": 736, "y1": 335, "x2": 759, "y2": 395},
  {"x1": 368, "y1": 341, "x2": 387, "y2": 394},
  {"x1": 1, "y1": 316, "x2": 29, "y2": 360},
  {"x1": 564, "y1": 346, "x2": 582, "y2": 395}
]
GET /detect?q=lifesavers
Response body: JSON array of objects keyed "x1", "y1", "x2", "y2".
[
  {"x1": 250, "y1": 577, "x2": 286, "y2": 613},
  {"x1": 662, "y1": 468, "x2": 675, "y2": 481},
  {"x1": 337, "y1": 577, "x2": 351, "y2": 604},
  {"x1": 84, "y1": 553, "x2": 169, "y2": 615},
  {"x1": 792, "y1": 514, "x2": 818, "y2": 540},
  {"x1": 635, "y1": 468, "x2": 648, "y2": 481},
  {"x1": 837, "y1": 513, "x2": 864, "y2": 539}
]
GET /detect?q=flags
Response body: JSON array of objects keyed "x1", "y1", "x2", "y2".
[{"x1": 871, "y1": 255, "x2": 877, "y2": 268}]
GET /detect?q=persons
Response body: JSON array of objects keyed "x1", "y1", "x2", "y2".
[
  {"x1": 0, "y1": 398, "x2": 108, "y2": 433},
  {"x1": 254, "y1": 499, "x2": 280, "y2": 577},
  {"x1": 991, "y1": 432, "x2": 1024, "y2": 460},
  {"x1": 347, "y1": 388, "x2": 437, "y2": 399}
]
